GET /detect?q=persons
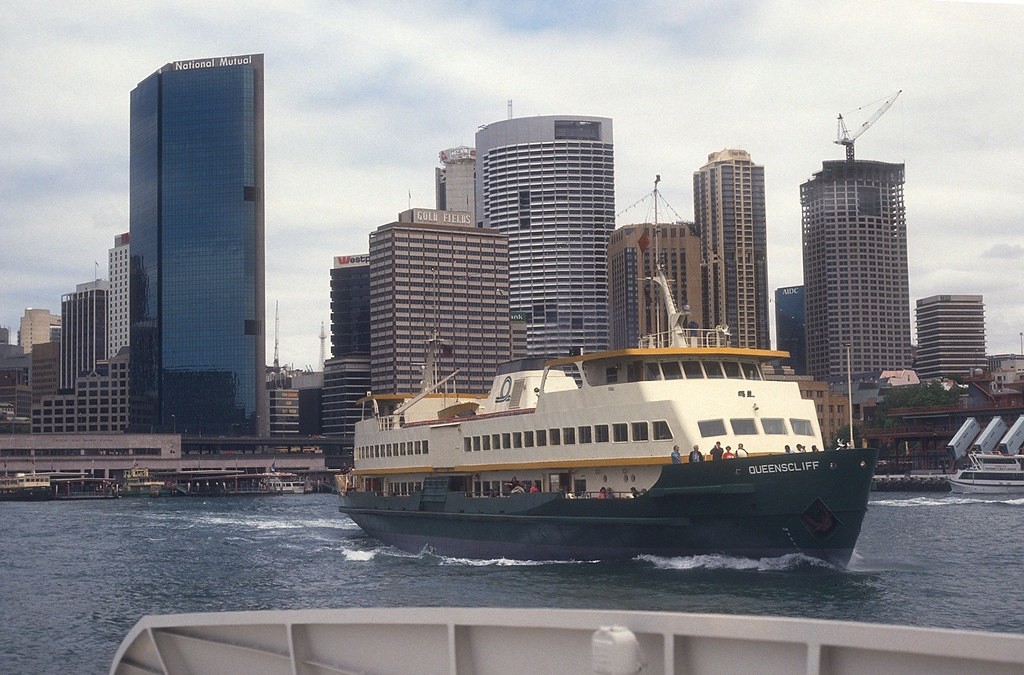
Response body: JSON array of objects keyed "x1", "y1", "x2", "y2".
[
  {"x1": 504, "y1": 476, "x2": 540, "y2": 494},
  {"x1": 785, "y1": 444, "x2": 818, "y2": 454},
  {"x1": 566, "y1": 487, "x2": 647, "y2": 499},
  {"x1": 671, "y1": 442, "x2": 749, "y2": 464}
]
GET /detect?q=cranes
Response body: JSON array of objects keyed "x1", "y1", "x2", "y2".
[{"x1": 834, "y1": 87, "x2": 901, "y2": 164}]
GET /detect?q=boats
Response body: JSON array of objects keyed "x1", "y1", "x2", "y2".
[
  {"x1": 338, "y1": 175, "x2": 882, "y2": 569},
  {"x1": 257, "y1": 472, "x2": 308, "y2": 493},
  {"x1": 945, "y1": 414, "x2": 1024, "y2": 504}
]
